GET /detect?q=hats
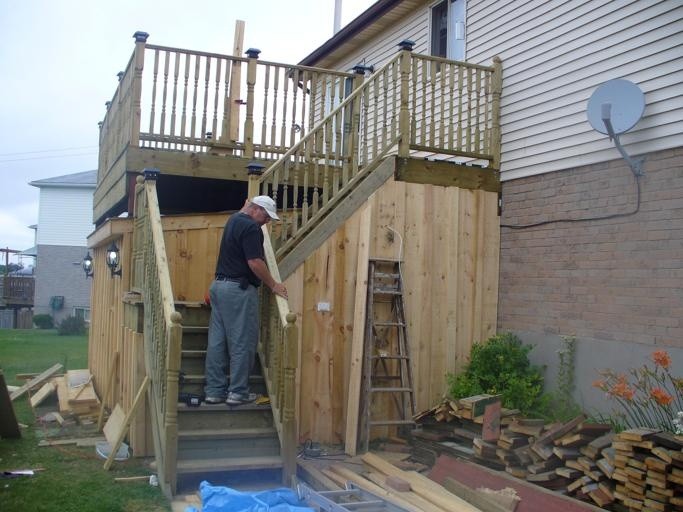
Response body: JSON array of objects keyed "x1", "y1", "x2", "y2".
[{"x1": 253, "y1": 195, "x2": 281, "y2": 222}]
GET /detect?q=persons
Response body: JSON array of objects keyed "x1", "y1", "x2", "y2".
[{"x1": 202, "y1": 194, "x2": 290, "y2": 405}]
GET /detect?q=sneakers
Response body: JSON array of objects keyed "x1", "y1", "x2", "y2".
[
  {"x1": 204, "y1": 395, "x2": 226, "y2": 405},
  {"x1": 226, "y1": 391, "x2": 257, "y2": 405}
]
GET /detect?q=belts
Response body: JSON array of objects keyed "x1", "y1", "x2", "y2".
[{"x1": 215, "y1": 275, "x2": 243, "y2": 283}]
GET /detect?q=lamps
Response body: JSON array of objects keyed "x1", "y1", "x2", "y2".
[
  {"x1": 106, "y1": 240, "x2": 121, "y2": 279},
  {"x1": 82, "y1": 251, "x2": 93, "y2": 279}
]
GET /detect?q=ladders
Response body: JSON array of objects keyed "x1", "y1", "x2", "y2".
[{"x1": 358, "y1": 258, "x2": 419, "y2": 455}]
